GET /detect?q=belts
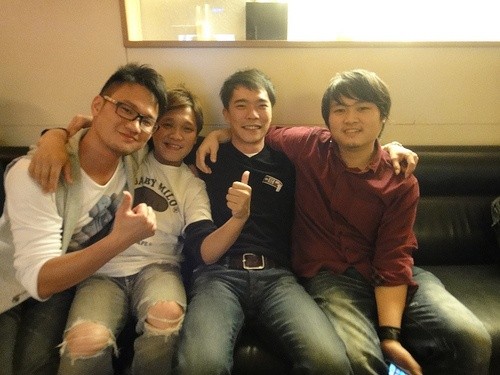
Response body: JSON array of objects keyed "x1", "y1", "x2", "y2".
[{"x1": 219, "y1": 253, "x2": 280, "y2": 270}]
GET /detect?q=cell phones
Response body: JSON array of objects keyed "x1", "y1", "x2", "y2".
[{"x1": 388, "y1": 362, "x2": 413, "y2": 375}]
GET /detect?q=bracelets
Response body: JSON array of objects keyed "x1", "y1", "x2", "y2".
[
  {"x1": 376, "y1": 325, "x2": 402, "y2": 342},
  {"x1": 385, "y1": 141, "x2": 404, "y2": 153},
  {"x1": 40, "y1": 127, "x2": 69, "y2": 144}
]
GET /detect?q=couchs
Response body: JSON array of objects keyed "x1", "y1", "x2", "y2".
[{"x1": 0, "y1": 145, "x2": 500, "y2": 375}]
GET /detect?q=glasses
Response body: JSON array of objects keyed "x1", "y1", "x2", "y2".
[{"x1": 103, "y1": 95, "x2": 160, "y2": 135}]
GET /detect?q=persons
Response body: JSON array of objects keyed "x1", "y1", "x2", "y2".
[{"x1": 0, "y1": 62, "x2": 492, "y2": 375}]
